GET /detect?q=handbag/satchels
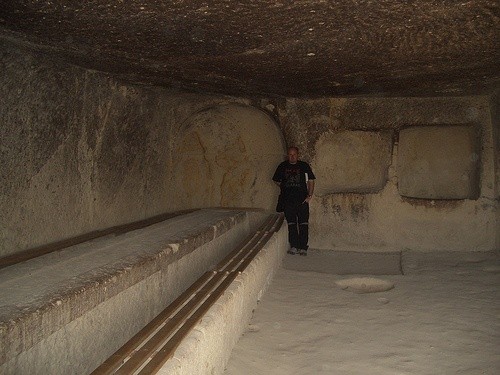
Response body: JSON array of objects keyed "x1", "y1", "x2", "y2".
[{"x1": 276, "y1": 193, "x2": 285, "y2": 213}]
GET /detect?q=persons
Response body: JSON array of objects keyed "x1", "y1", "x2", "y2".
[{"x1": 273, "y1": 147, "x2": 317, "y2": 255}]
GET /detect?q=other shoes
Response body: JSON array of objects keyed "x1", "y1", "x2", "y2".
[{"x1": 288, "y1": 247, "x2": 308, "y2": 255}]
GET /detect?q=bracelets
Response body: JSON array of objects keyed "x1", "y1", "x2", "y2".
[{"x1": 307, "y1": 194, "x2": 312, "y2": 199}]
